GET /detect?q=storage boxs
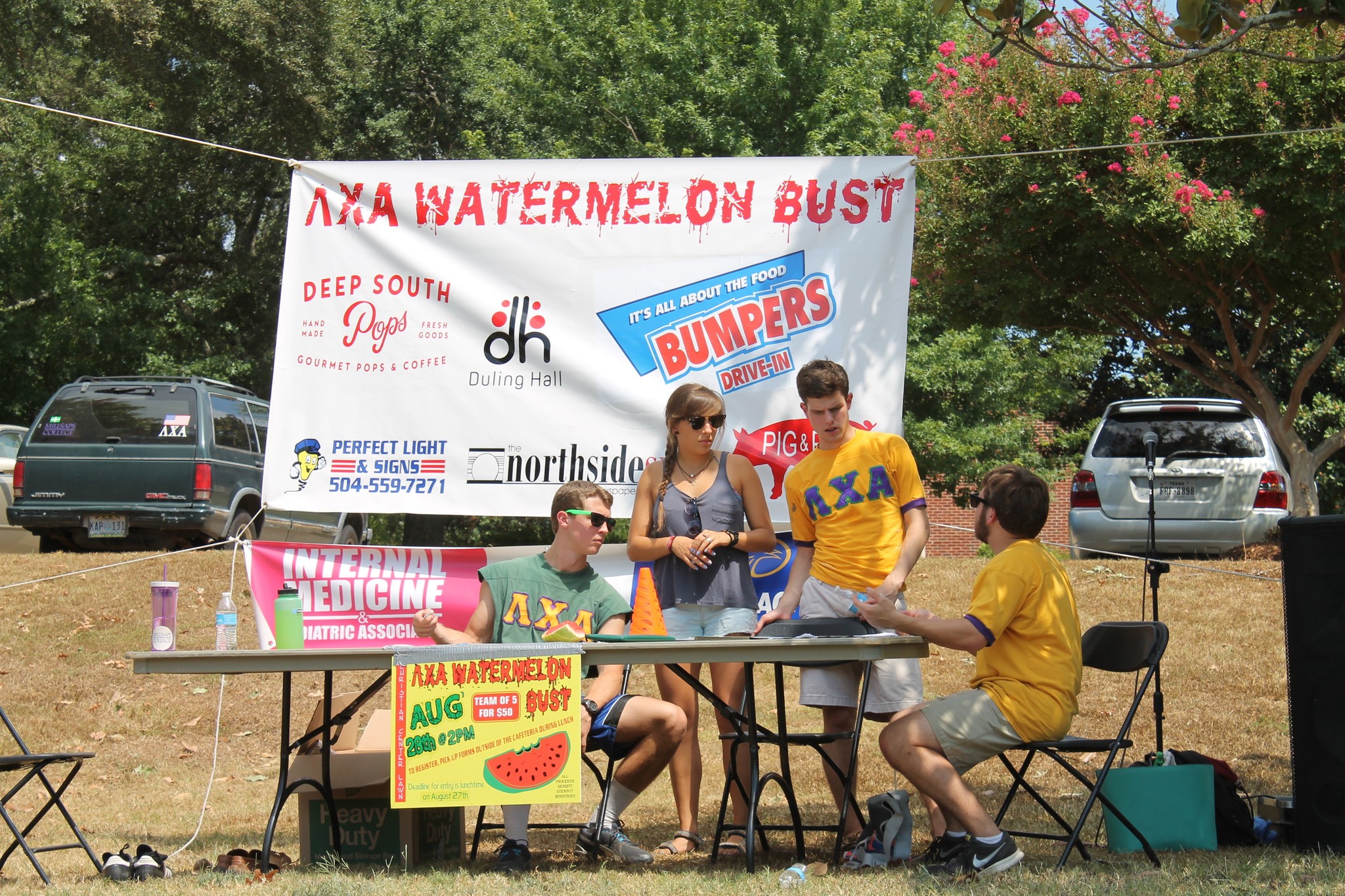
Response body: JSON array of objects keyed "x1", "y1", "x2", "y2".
[{"x1": 286, "y1": 691, "x2": 466, "y2": 876}]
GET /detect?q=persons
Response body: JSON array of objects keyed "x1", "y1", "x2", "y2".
[
  {"x1": 411, "y1": 481, "x2": 690, "y2": 873},
  {"x1": 852, "y1": 465, "x2": 1083, "y2": 879},
  {"x1": 749, "y1": 359, "x2": 947, "y2": 868},
  {"x1": 626, "y1": 384, "x2": 778, "y2": 862}
]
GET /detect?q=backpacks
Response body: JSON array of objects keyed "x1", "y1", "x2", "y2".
[
  {"x1": 848, "y1": 789, "x2": 913, "y2": 869},
  {"x1": 1129, "y1": 748, "x2": 1261, "y2": 847}
]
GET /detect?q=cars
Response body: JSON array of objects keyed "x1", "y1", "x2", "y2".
[{"x1": 0, "y1": 424, "x2": 29, "y2": 530}]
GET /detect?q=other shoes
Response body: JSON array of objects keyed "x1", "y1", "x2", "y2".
[
  {"x1": 842, "y1": 831, "x2": 863, "y2": 853},
  {"x1": 217, "y1": 848, "x2": 281, "y2": 874},
  {"x1": 900, "y1": 853, "x2": 925, "y2": 868}
]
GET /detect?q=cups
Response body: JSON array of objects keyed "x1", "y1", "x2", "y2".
[{"x1": 149, "y1": 581, "x2": 180, "y2": 652}]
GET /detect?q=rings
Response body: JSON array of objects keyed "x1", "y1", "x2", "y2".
[
  {"x1": 702, "y1": 534, "x2": 706, "y2": 538},
  {"x1": 689, "y1": 554, "x2": 694, "y2": 559},
  {"x1": 707, "y1": 538, "x2": 712, "y2": 542}
]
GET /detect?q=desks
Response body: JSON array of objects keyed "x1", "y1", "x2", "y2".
[{"x1": 125, "y1": 636, "x2": 932, "y2": 872}]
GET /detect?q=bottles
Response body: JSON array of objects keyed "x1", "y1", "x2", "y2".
[
  {"x1": 215, "y1": 593, "x2": 238, "y2": 651},
  {"x1": 831, "y1": 586, "x2": 868, "y2": 618},
  {"x1": 780, "y1": 863, "x2": 809, "y2": 889},
  {"x1": 275, "y1": 583, "x2": 304, "y2": 650}
]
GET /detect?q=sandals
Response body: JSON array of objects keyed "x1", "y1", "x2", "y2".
[
  {"x1": 718, "y1": 829, "x2": 760, "y2": 861},
  {"x1": 654, "y1": 829, "x2": 702, "y2": 857}
]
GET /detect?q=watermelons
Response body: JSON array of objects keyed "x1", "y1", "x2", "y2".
[{"x1": 541, "y1": 620, "x2": 586, "y2": 642}]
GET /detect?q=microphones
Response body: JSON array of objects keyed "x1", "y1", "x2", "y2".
[{"x1": 1143, "y1": 431, "x2": 1158, "y2": 495}]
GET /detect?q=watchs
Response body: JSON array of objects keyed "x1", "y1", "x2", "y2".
[
  {"x1": 727, "y1": 528, "x2": 739, "y2": 547},
  {"x1": 581, "y1": 699, "x2": 600, "y2": 717}
]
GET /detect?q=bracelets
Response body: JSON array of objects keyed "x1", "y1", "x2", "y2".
[
  {"x1": 667, "y1": 535, "x2": 677, "y2": 554},
  {"x1": 721, "y1": 530, "x2": 732, "y2": 546}
]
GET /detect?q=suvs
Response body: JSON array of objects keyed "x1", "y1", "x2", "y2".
[
  {"x1": 1067, "y1": 397, "x2": 1319, "y2": 562},
  {"x1": 6, "y1": 373, "x2": 373, "y2": 552}
]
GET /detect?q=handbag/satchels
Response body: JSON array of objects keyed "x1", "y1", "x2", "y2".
[{"x1": 1094, "y1": 763, "x2": 1219, "y2": 852}]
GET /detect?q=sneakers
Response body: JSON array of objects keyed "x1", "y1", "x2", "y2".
[
  {"x1": 573, "y1": 819, "x2": 654, "y2": 866},
  {"x1": 101, "y1": 843, "x2": 134, "y2": 882},
  {"x1": 133, "y1": 843, "x2": 175, "y2": 882},
  {"x1": 907, "y1": 831, "x2": 970, "y2": 873},
  {"x1": 910, "y1": 829, "x2": 1025, "y2": 894},
  {"x1": 492, "y1": 835, "x2": 533, "y2": 877}
]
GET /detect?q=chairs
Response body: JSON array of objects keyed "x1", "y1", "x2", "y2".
[
  {"x1": 0, "y1": 707, "x2": 103, "y2": 885},
  {"x1": 467, "y1": 664, "x2": 630, "y2": 862},
  {"x1": 711, "y1": 617, "x2": 868, "y2": 873},
  {"x1": 994, "y1": 622, "x2": 1170, "y2": 873}
]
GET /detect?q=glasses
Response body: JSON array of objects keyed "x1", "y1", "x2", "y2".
[
  {"x1": 969, "y1": 492, "x2": 988, "y2": 509},
  {"x1": 684, "y1": 497, "x2": 703, "y2": 536},
  {"x1": 679, "y1": 413, "x2": 726, "y2": 431},
  {"x1": 566, "y1": 509, "x2": 616, "y2": 533}
]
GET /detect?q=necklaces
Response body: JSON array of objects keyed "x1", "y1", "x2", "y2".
[{"x1": 675, "y1": 452, "x2": 714, "y2": 486}]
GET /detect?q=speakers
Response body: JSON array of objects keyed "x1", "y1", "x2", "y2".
[{"x1": 1275, "y1": 515, "x2": 1345, "y2": 856}]
